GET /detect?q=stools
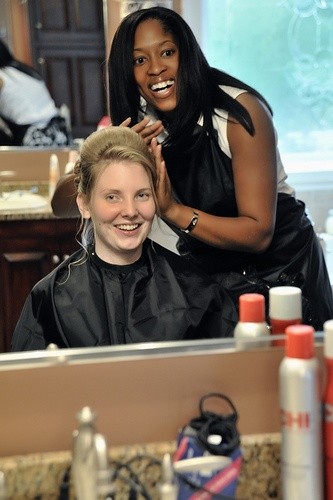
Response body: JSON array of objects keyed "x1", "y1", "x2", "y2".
[{"x1": 4, "y1": 252, "x2": 44, "y2": 352}]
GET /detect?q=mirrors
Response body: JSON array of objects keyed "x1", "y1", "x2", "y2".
[{"x1": 0, "y1": 0, "x2": 333, "y2": 371}]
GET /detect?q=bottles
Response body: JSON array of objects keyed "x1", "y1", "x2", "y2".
[
  {"x1": 234, "y1": 294, "x2": 271, "y2": 350},
  {"x1": 277, "y1": 325, "x2": 323, "y2": 500},
  {"x1": 323, "y1": 320, "x2": 333, "y2": 500},
  {"x1": 268, "y1": 285, "x2": 303, "y2": 346}
]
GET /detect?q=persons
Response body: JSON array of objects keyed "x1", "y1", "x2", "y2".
[
  {"x1": 0, "y1": 38, "x2": 70, "y2": 147},
  {"x1": 108, "y1": 6, "x2": 333, "y2": 332},
  {"x1": 9, "y1": 126, "x2": 240, "y2": 353}
]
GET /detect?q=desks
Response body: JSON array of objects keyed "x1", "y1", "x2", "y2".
[
  {"x1": 0, "y1": 432, "x2": 333, "y2": 500},
  {"x1": 0, "y1": 178, "x2": 89, "y2": 356}
]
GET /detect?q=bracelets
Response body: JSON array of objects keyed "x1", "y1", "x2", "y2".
[{"x1": 181, "y1": 209, "x2": 199, "y2": 233}]
